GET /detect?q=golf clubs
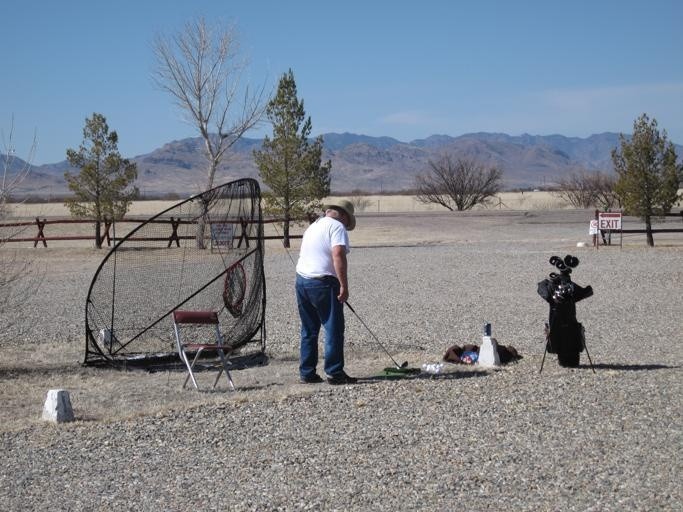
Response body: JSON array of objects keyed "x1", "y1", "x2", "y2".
[
  {"x1": 549, "y1": 254, "x2": 579, "y2": 302},
  {"x1": 345, "y1": 301, "x2": 407, "y2": 369}
]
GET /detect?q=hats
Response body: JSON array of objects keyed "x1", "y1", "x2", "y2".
[{"x1": 321, "y1": 196, "x2": 355, "y2": 231}]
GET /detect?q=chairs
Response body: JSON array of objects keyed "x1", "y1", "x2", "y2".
[{"x1": 172, "y1": 309, "x2": 237, "y2": 393}]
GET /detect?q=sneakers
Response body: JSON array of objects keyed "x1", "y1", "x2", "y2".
[
  {"x1": 299, "y1": 375, "x2": 322, "y2": 384},
  {"x1": 327, "y1": 372, "x2": 357, "y2": 385}
]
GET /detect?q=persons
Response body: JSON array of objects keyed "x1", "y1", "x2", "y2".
[{"x1": 294, "y1": 199, "x2": 358, "y2": 385}]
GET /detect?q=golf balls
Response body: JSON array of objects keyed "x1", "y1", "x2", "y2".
[{"x1": 423, "y1": 363, "x2": 444, "y2": 373}]
